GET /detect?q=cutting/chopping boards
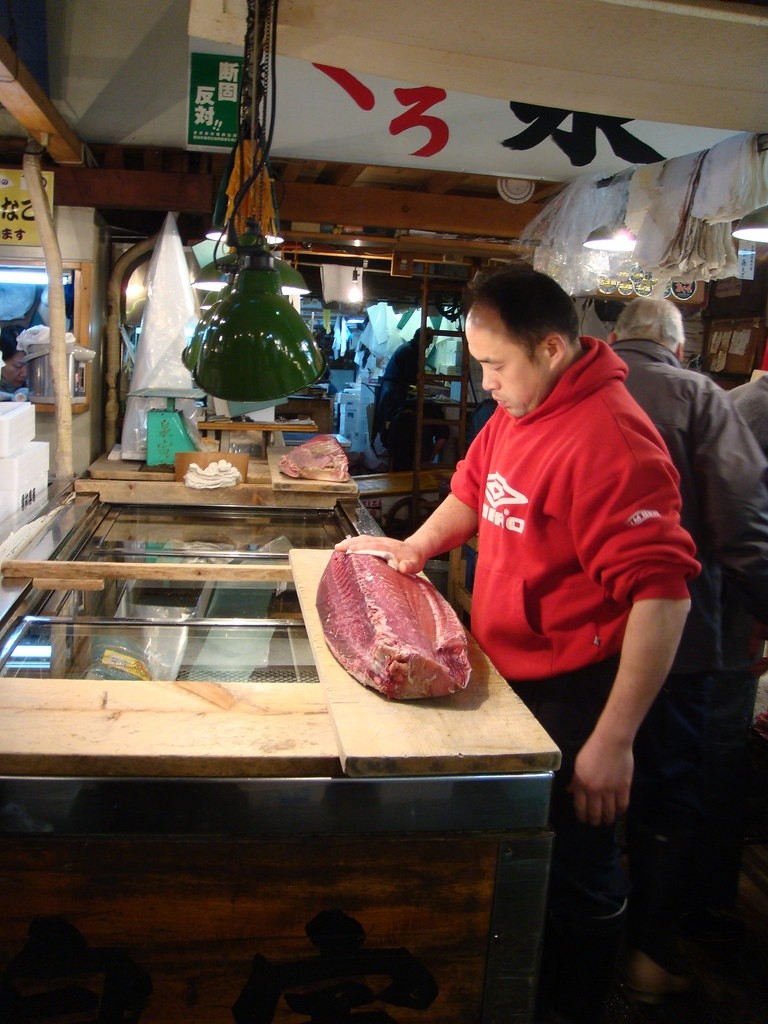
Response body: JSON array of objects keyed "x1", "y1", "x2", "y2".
[
  {"x1": 265, "y1": 446, "x2": 358, "y2": 495},
  {"x1": 288, "y1": 545, "x2": 562, "y2": 775}
]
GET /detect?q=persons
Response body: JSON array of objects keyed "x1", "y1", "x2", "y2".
[
  {"x1": 335, "y1": 263, "x2": 768, "y2": 996},
  {"x1": 0, "y1": 325, "x2": 32, "y2": 394}
]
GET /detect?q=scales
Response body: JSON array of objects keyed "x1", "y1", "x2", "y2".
[{"x1": 126, "y1": 386, "x2": 208, "y2": 467}]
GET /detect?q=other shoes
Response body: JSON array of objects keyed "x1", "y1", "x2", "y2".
[
  {"x1": 613, "y1": 946, "x2": 693, "y2": 1007},
  {"x1": 552, "y1": 877, "x2": 628, "y2": 921}
]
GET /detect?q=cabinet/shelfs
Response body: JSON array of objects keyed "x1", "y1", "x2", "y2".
[{"x1": 278, "y1": 395, "x2": 333, "y2": 434}]
